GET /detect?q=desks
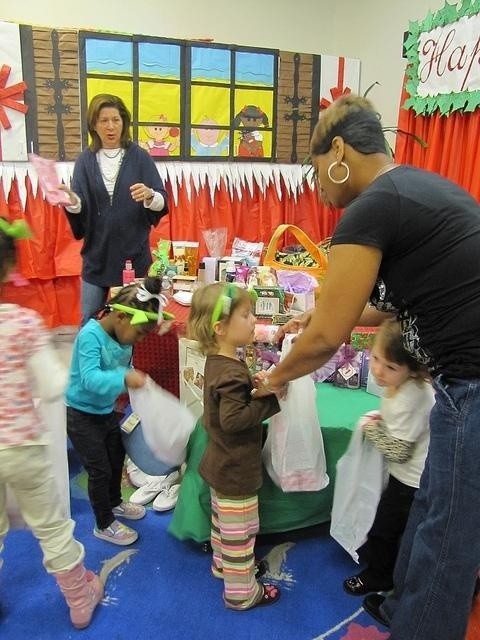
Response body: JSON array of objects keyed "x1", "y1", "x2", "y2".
[
  {"x1": 168, "y1": 383, "x2": 391, "y2": 553},
  {"x1": 107, "y1": 267, "x2": 384, "y2": 399}
]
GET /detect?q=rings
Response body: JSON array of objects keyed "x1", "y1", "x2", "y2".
[{"x1": 142, "y1": 192, "x2": 145, "y2": 197}]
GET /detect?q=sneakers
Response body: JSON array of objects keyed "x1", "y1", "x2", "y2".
[
  {"x1": 92, "y1": 518, "x2": 139, "y2": 547},
  {"x1": 112, "y1": 501, "x2": 146, "y2": 521},
  {"x1": 210, "y1": 559, "x2": 271, "y2": 584},
  {"x1": 128, "y1": 470, "x2": 182, "y2": 514},
  {"x1": 252, "y1": 575, "x2": 281, "y2": 608}
]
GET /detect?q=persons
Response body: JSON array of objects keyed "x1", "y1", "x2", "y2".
[
  {"x1": 57, "y1": 94, "x2": 171, "y2": 330},
  {"x1": 183, "y1": 280, "x2": 283, "y2": 612},
  {"x1": 343, "y1": 315, "x2": 438, "y2": 598},
  {"x1": 242, "y1": 89, "x2": 480, "y2": 639},
  {"x1": 63, "y1": 275, "x2": 165, "y2": 547},
  {"x1": 0, "y1": 216, "x2": 105, "y2": 630}
]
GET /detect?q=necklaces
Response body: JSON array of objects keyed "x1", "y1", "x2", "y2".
[
  {"x1": 95, "y1": 149, "x2": 125, "y2": 182},
  {"x1": 100, "y1": 146, "x2": 121, "y2": 159},
  {"x1": 370, "y1": 160, "x2": 394, "y2": 184}
]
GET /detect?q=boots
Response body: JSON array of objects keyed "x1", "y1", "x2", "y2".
[{"x1": 42, "y1": 540, "x2": 105, "y2": 632}]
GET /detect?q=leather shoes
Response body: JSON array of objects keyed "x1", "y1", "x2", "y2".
[{"x1": 342, "y1": 566, "x2": 395, "y2": 631}]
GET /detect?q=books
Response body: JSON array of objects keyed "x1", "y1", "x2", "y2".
[{"x1": 27, "y1": 153, "x2": 75, "y2": 209}]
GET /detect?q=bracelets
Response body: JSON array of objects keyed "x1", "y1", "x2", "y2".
[
  {"x1": 146, "y1": 187, "x2": 155, "y2": 201},
  {"x1": 261, "y1": 372, "x2": 288, "y2": 397}
]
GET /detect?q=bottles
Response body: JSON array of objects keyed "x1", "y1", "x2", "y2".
[
  {"x1": 197, "y1": 263, "x2": 206, "y2": 282},
  {"x1": 123, "y1": 259, "x2": 136, "y2": 286}
]
